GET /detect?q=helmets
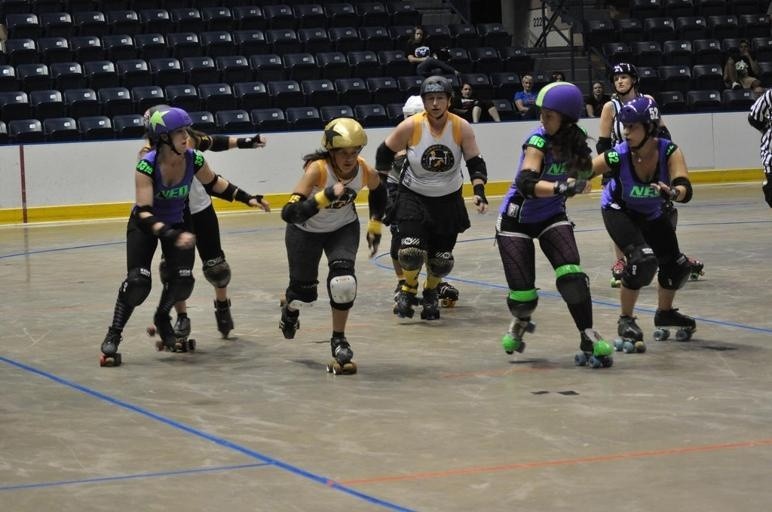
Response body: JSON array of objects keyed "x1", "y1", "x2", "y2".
[
  {"x1": 402, "y1": 76, "x2": 452, "y2": 120},
  {"x1": 322, "y1": 118, "x2": 367, "y2": 150},
  {"x1": 143, "y1": 104, "x2": 192, "y2": 137},
  {"x1": 536, "y1": 81, "x2": 583, "y2": 121},
  {"x1": 610, "y1": 63, "x2": 659, "y2": 122}
]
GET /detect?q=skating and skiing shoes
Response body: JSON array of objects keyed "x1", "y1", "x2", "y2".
[
  {"x1": 279, "y1": 293, "x2": 303, "y2": 339},
  {"x1": 100, "y1": 326, "x2": 123, "y2": 366},
  {"x1": 688, "y1": 255, "x2": 704, "y2": 279},
  {"x1": 147, "y1": 306, "x2": 196, "y2": 351},
  {"x1": 610, "y1": 258, "x2": 626, "y2": 287},
  {"x1": 393, "y1": 279, "x2": 458, "y2": 320},
  {"x1": 503, "y1": 316, "x2": 534, "y2": 354},
  {"x1": 575, "y1": 307, "x2": 696, "y2": 368},
  {"x1": 326, "y1": 337, "x2": 357, "y2": 374},
  {"x1": 214, "y1": 299, "x2": 234, "y2": 339}
]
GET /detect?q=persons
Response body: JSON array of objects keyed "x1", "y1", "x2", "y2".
[
  {"x1": 102, "y1": 108, "x2": 271, "y2": 354},
  {"x1": 279, "y1": 118, "x2": 388, "y2": 362},
  {"x1": 366, "y1": 76, "x2": 489, "y2": 320},
  {"x1": 495, "y1": 62, "x2": 706, "y2": 353},
  {"x1": 723, "y1": 41, "x2": 766, "y2": 94},
  {"x1": 403, "y1": 24, "x2": 571, "y2": 123},
  {"x1": 136, "y1": 103, "x2": 266, "y2": 337},
  {"x1": 747, "y1": 87, "x2": 772, "y2": 206}
]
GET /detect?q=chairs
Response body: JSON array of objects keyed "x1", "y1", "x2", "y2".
[
  {"x1": 568, "y1": 1, "x2": 771, "y2": 114},
  {"x1": 0, "y1": 0, "x2": 554, "y2": 139}
]
